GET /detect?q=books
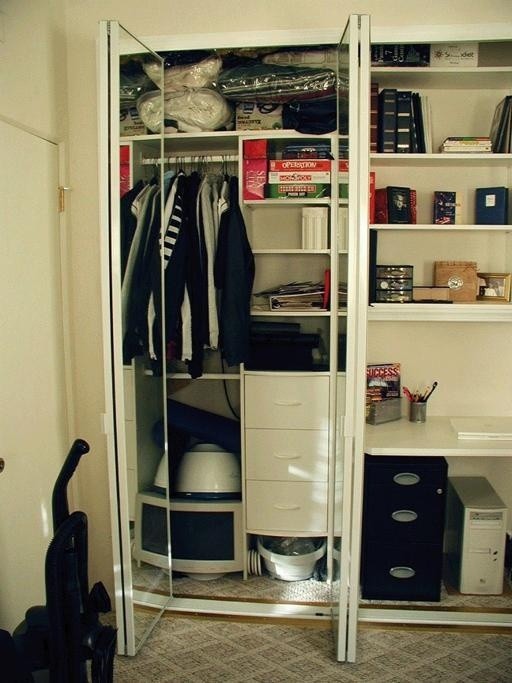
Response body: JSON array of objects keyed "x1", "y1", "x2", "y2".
[
  {"x1": 441, "y1": 95, "x2": 511, "y2": 153},
  {"x1": 373, "y1": 80, "x2": 431, "y2": 153}
]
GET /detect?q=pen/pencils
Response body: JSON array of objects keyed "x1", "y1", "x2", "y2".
[{"x1": 402, "y1": 382, "x2": 438, "y2": 403}]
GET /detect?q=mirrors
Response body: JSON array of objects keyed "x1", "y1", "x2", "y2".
[
  {"x1": 327, "y1": 14, "x2": 360, "y2": 661},
  {"x1": 110, "y1": 20, "x2": 176, "y2": 658}
]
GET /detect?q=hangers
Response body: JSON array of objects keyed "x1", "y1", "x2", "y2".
[{"x1": 142, "y1": 154, "x2": 237, "y2": 184}]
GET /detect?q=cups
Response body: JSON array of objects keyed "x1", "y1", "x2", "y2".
[{"x1": 409, "y1": 401, "x2": 427, "y2": 423}]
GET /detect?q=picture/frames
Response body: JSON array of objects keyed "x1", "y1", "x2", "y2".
[{"x1": 386, "y1": 186, "x2": 413, "y2": 224}]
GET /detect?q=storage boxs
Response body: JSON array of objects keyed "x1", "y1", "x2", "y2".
[
  {"x1": 243, "y1": 139, "x2": 276, "y2": 200},
  {"x1": 428, "y1": 43, "x2": 479, "y2": 66},
  {"x1": 236, "y1": 101, "x2": 283, "y2": 131},
  {"x1": 370, "y1": 43, "x2": 430, "y2": 67}
]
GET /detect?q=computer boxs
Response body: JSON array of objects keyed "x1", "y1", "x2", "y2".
[{"x1": 444, "y1": 476, "x2": 510, "y2": 595}]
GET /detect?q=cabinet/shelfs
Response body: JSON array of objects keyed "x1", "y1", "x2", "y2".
[
  {"x1": 92, "y1": 14, "x2": 359, "y2": 662},
  {"x1": 346, "y1": 15, "x2": 510, "y2": 663}
]
